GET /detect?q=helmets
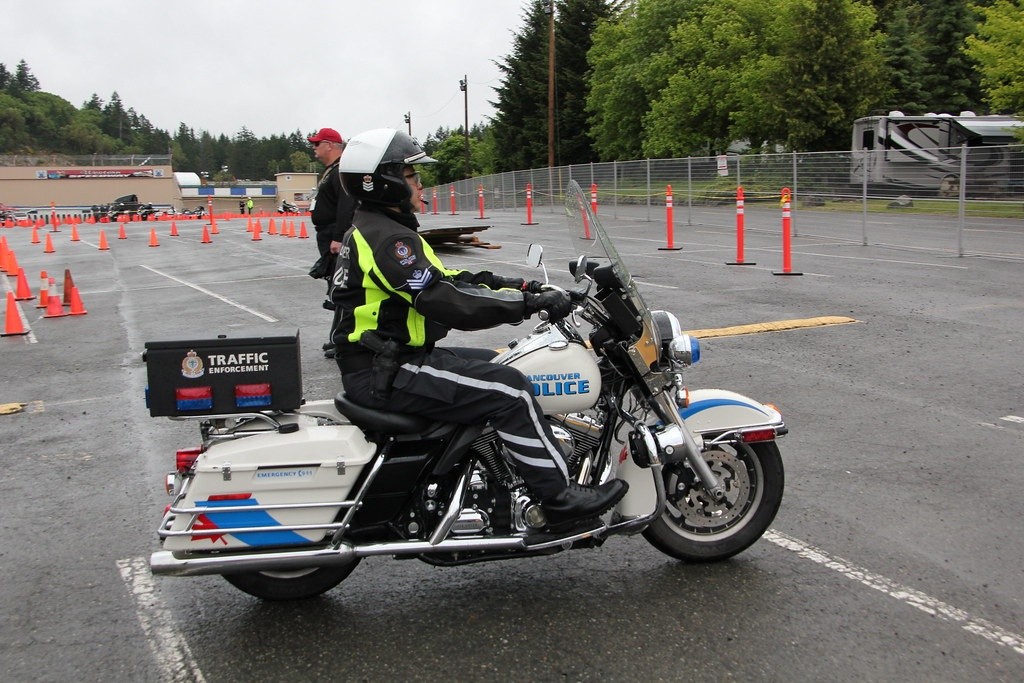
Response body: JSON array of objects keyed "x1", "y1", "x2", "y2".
[
  {"x1": 247, "y1": 197, "x2": 251, "y2": 199},
  {"x1": 148, "y1": 202, "x2": 152, "y2": 204},
  {"x1": 282, "y1": 199, "x2": 286, "y2": 201},
  {"x1": 119, "y1": 203, "x2": 124, "y2": 205},
  {"x1": 339, "y1": 128, "x2": 439, "y2": 206}
]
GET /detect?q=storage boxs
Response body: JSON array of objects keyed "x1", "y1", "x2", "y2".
[
  {"x1": 163, "y1": 424, "x2": 378, "y2": 550},
  {"x1": 140, "y1": 326, "x2": 303, "y2": 416}
]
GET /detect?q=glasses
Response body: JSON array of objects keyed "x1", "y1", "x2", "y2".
[
  {"x1": 405, "y1": 171, "x2": 420, "y2": 185},
  {"x1": 310, "y1": 141, "x2": 330, "y2": 146}
]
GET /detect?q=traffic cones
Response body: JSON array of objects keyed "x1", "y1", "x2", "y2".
[
  {"x1": 115, "y1": 211, "x2": 310, "y2": 248},
  {"x1": 1, "y1": 211, "x2": 110, "y2": 254},
  {"x1": 0, "y1": 233, "x2": 86, "y2": 339}
]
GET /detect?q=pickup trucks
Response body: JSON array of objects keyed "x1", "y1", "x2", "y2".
[{"x1": 92, "y1": 194, "x2": 155, "y2": 223}]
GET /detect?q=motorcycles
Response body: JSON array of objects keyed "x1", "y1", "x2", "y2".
[{"x1": 142, "y1": 128, "x2": 783, "y2": 604}]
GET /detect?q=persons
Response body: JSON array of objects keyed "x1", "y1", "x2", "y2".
[
  {"x1": 308, "y1": 128, "x2": 357, "y2": 359},
  {"x1": 106, "y1": 203, "x2": 110, "y2": 212},
  {"x1": 109, "y1": 203, "x2": 114, "y2": 211},
  {"x1": 100, "y1": 204, "x2": 106, "y2": 216},
  {"x1": 282, "y1": 199, "x2": 296, "y2": 212},
  {"x1": 140, "y1": 202, "x2": 200, "y2": 216},
  {"x1": 329, "y1": 127, "x2": 630, "y2": 534},
  {"x1": 239, "y1": 197, "x2": 245, "y2": 214},
  {"x1": 246, "y1": 196, "x2": 254, "y2": 215},
  {"x1": 91, "y1": 205, "x2": 99, "y2": 222}
]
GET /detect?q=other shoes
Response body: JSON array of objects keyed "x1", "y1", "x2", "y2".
[{"x1": 322, "y1": 342, "x2": 336, "y2": 358}]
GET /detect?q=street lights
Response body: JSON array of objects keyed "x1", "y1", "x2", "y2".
[
  {"x1": 403, "y1": 110, "x2": 411, "y2": 135},
  {"x1": 459, "y1": 73, "x2": 469, "y2": 177}
]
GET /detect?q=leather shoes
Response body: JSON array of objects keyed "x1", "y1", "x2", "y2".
[{"x1": 543, "y1": 478, "x2": 630, "y2": 533}]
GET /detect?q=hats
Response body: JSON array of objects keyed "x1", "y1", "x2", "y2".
[{"x1": 307, "y1": 128, "x2": 342, "y2": 143}]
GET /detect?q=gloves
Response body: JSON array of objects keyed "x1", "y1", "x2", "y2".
[
  {"x1": 499, "y1": 277, "x2": 525, "y2": 290},
  {"x1": 524, "y1": 290, "x2": 572, "y2": 325}
]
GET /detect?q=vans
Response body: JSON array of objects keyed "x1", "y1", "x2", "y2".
[{"x1": 850, "y1": 108, "x2": 1023, "y2": 201}]
